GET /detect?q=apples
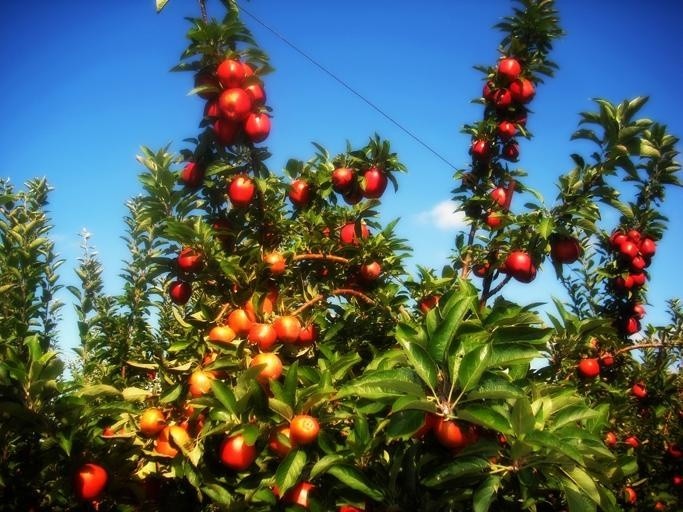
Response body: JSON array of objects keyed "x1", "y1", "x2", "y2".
[
  {"x1": 103, "y1": 55, "x2": 388, "y2": 512},
  {"x1": 416, "y1": 58, "x2": 683, "y2": 512},
  {"x1": 73, "y1": 462, "x2": 109, "y2": 503}
]
